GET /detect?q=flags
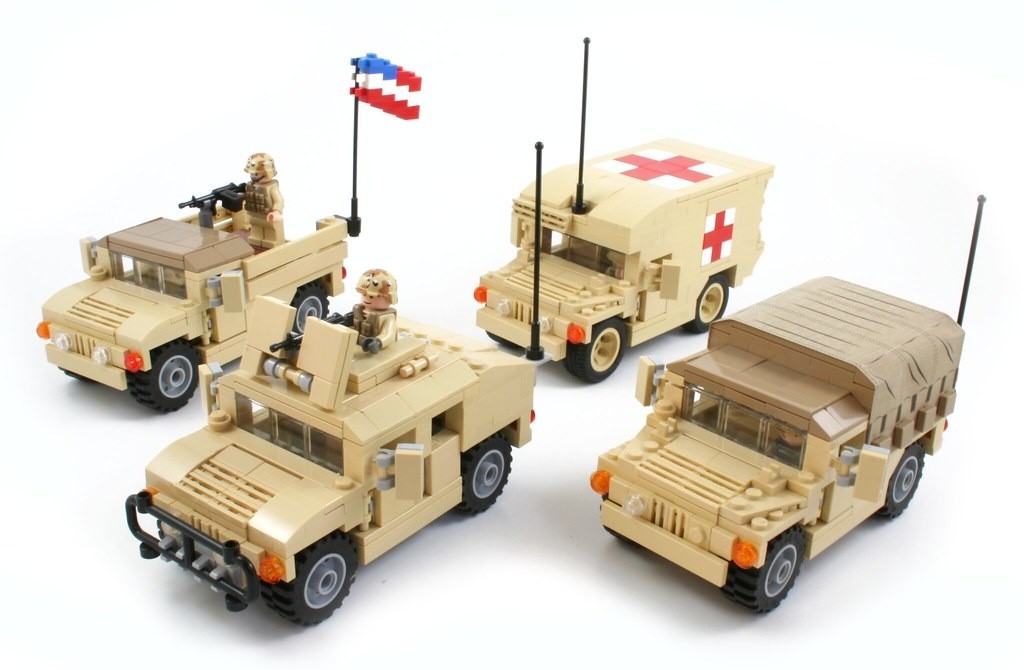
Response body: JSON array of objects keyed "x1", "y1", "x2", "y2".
[{"x1": 352, "y1": 53, "x2": 423, "y2": 122}]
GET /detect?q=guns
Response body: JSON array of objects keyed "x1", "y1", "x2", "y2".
[
  {"x1": 179, "y1": 182, "x2": 247, "y2": 218},
  {"x1": 269, "y1": 311, "x2": 355, "y2": 352}
]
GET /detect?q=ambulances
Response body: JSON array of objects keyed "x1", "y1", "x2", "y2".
[{"x1": 473, "y1": 37, "x2": 775, "y2": 383}]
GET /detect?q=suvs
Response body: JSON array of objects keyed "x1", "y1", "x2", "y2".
[
  {"x1": 125, "y1": 269, "x2": 538, "y2": 626},
  {"x1": 37, "y1": 51, "x2": 422, "y2": 414},
  {"x1": 588, "y1": 194, "x2": 987, "y2": 615}
]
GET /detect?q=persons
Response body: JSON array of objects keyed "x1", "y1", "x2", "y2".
[
  {"x1": 765, "y1": 419, "x2": 808, "y2": 469},
  {"x1": 602, "y1": 248, "x2": 626, "y2": 281},
  {"x1": 239, "y1": 154, "x2": 285, "y2": 247},
  {"x1": 332, "y1": 270, "x2": 399, "y2": 354}
]
